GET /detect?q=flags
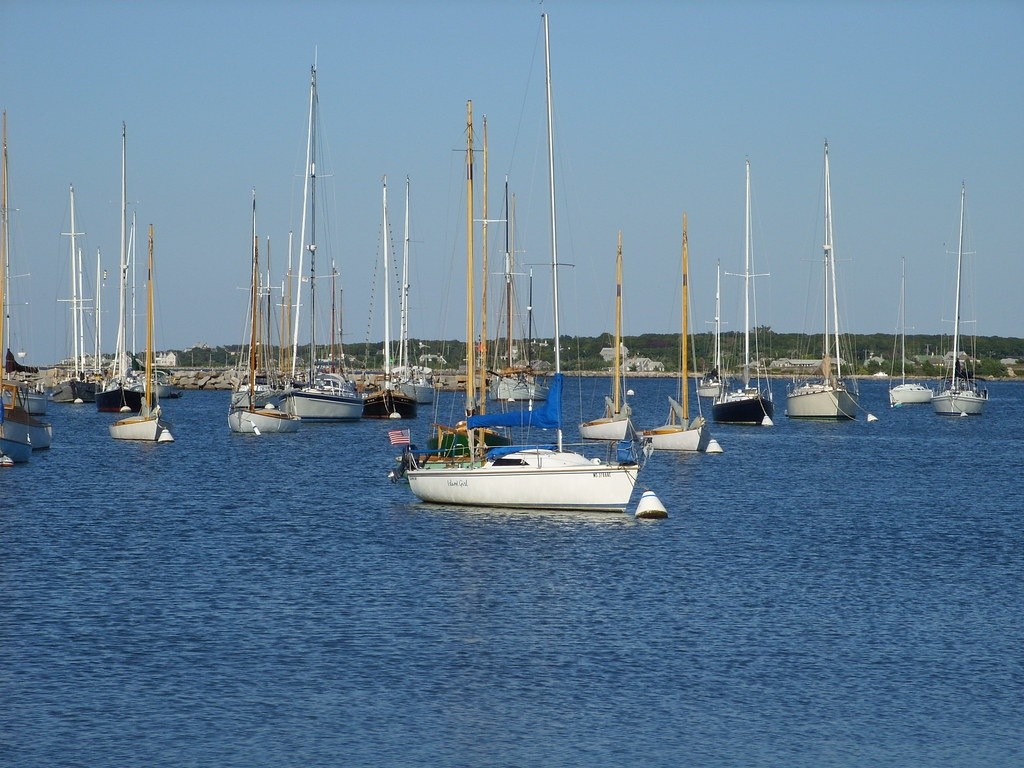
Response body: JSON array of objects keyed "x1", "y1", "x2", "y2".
[{"x1": 388, "y1": 429, "x2": 410, "y2": 445}]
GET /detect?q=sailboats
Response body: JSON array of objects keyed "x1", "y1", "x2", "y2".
[
  {"x1": 406, "y1": 91, "x2": 668, "y2": 523},
  {"x1": 0, "y1": 10, "x2": 986, "y2": 455}
]
